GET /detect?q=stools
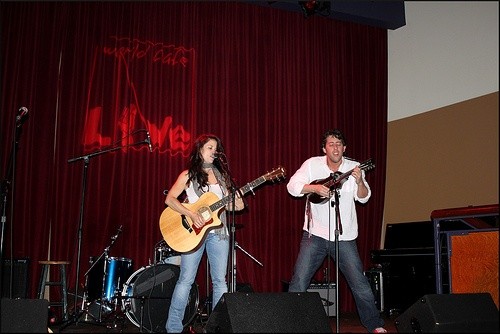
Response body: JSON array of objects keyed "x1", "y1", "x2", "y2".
[{"x1": 35, "y1": 260, "x2": 70, "y2": 320}]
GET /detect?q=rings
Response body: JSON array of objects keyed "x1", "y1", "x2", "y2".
[{"x1": 199, "y1": 222, "x2": 202, "y2": 225}]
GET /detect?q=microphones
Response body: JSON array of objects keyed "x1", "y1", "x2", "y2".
[
  {"x1": 211, "y1": 151, "x2": 225, "y2": 158},
  {"x1": 145, "y1": 132, "x2": 153, "y2": 152},
  {"x1": 16, "y1": 106, "x2": 28, "y2": 120}
]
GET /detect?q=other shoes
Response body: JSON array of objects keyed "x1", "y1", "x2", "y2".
[{"x1": 371, "y1": 327, "x2": 387, "y2": 334}]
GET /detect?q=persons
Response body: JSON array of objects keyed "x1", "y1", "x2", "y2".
[
  {"x1": 286, "y1": 129, "x2": 388, "y2": 334},
  {"x1": 164, "y1": 134, "x2": 245, "y2": 334}
]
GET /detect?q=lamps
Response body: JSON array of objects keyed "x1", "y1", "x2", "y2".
[{"x1": 297, "y1": 0, "x2": 332, "y2": 19}]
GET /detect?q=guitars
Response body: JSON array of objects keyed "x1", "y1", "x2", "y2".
[
  {"x1": 159, "y1": 164, "x2": 288, "y2": 255},
  {"x1": 305, "y1": 158, "x2": 376, "y2": 204}
]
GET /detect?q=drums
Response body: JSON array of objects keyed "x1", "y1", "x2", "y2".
[
  {"x1": 155, "y1": 240, "x2": 182, "y2": 265},
  {"x1": 122, "y1": 262, "x2": 200, "y2": 334},
  {"x1": 88, "y1": 256, "x2": 134, "y2": 305}
]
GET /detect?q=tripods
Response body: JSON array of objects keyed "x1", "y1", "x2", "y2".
[{"x1": 53, "y1": 140, "x2": 144, "y2": 333}]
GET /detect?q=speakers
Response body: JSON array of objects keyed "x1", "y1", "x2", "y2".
[
  {"x1": 0, "y1": 298, "x2": 49, "y2": 334},
  {"x1": 377, "y1": 251, "x2": 450, "y2": 314},
  {"x1": 203, "y1": 292, "x2": 334, "y2": 334},
  {"x1": 395, "y1": 291, "x2": 500, "y2": 334},
  {"x1": 281, "y1": 281, "x2": 337, "y2": 317},
  {"x1": 0, "y1": 259, "x2": 29, "y2": 296}
]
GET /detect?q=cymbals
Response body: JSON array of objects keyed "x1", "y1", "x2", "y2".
[
  {"x1": 227, "y1": 223, "x2": 245, "y2": 230},
  {"x1": 67, "y1": 302, "x2": 112, "y2": 321}
]
